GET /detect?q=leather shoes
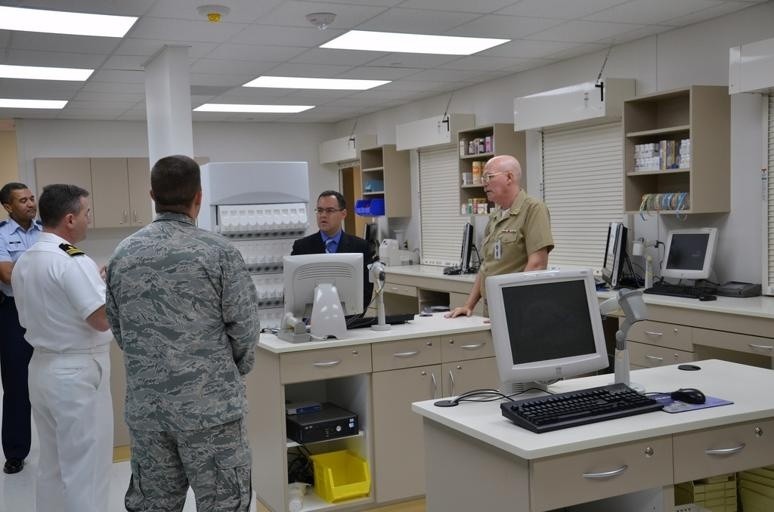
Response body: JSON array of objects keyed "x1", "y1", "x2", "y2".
[{"x1": 2, "y1": 457, "x2": 25, "y2": 476}]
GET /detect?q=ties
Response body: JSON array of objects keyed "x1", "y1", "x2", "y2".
[{"x1": 323, "y1": 236, "x2": 336, "y2": 254}]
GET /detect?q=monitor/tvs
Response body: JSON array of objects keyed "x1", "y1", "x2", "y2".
[
  {"x1": 282, "y1": 253, "x2": 366, "y2": 339},
  {"x1": 660, "y1": 227, "x2": 721, "y2": 285},
  {"x1": 485, "y1": 268, "x2": 610, "y2": 394},
  {"x1": 461, "y1": 222, "x2": 475, "y2": 274}
]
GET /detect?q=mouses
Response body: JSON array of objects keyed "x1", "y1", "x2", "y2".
[
  {"x1": 700, "y1": 295, "x2": 716, "y2": 301},
  {"x1": 673, "y1": 388, "x2": 706, "y2": 404},
  {"x1": 449, "y1": 271, "x2": 458, "y2": 275}
]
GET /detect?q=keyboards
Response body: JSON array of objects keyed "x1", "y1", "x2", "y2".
[
  {"x1": 443, "y1": 265, "x2": 461, "y2": 275},
  {"x1": 644, "y1": 284, "x2": 704, "y2": 297},
  {"x1": 346, "y1": 312, "x2": 415, "y2": 330},
  {"x1": 500, "y1": 382, "x2": 664, "y2": 433}
]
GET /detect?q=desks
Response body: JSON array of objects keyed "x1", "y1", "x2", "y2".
[
  {"x1": 409, "y1": 356, "x2": 774, "y2": 511},
  {"x1": 245, "y1": 307, "x2": 502, "y2": 511},
  {"x1": 361, "y1": 263, "x2": 772, "y2": 364}
]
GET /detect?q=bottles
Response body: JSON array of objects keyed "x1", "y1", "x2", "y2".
[
  {"x1": 473, "y1": 161, "x2": 482, "y2": 184},
  {"x1": 462, "y1": 173, "x2": 468, "y2": 186},
  {"x1": 461, "y1": 198, "x2": 487, "y2": 214},
  {"x1": 458, "y1": 134, "x2": 491, "y2": 155}
]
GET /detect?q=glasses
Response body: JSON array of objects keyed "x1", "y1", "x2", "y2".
[
  {"x1": 313, "y1": 206, "x2": 344, "y2": 213},
  {"x1": 481, "y1": 172, "x2": 515, "y2": 184}
]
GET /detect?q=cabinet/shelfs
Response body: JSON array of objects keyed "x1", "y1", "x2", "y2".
[
  {"x1": 89, "y1": 156, "x2": 153, "y2": 228},
  {"x1": 621, "y1": 84, "x2": 733, "y2": 222},
  {"x1": 35, "y1": 155, "x2": 93, "y2": 228},
  {"x1": 458, "y1": 118, "x2": 528, "y2": 221},
  {"x1": 362, "y1": 138, "x2": 413, "y2": 218},
  {"x1": 0, "y1": 117, "x2": 20, "y2": 223}
]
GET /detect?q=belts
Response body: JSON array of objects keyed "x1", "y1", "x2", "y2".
[{"x1": 30, "y1": 341, "x2": 112, "y2": 358}]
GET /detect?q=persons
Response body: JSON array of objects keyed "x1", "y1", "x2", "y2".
[
  {"x1": 0, "y1": 180, "x2": 46, "y2": 473},
  {"x1": 105, "y1": 155, "x2": 261, "y2": 512},
  {"x1": 443, "y1": 156, "x2": 554, "y2": 319},
  {"x1": 291, "y1": 189, "x2": 376, "y2": 318},
  {"x1": 10, "y1": 183, "x2": 115, "y2": 512}
]
